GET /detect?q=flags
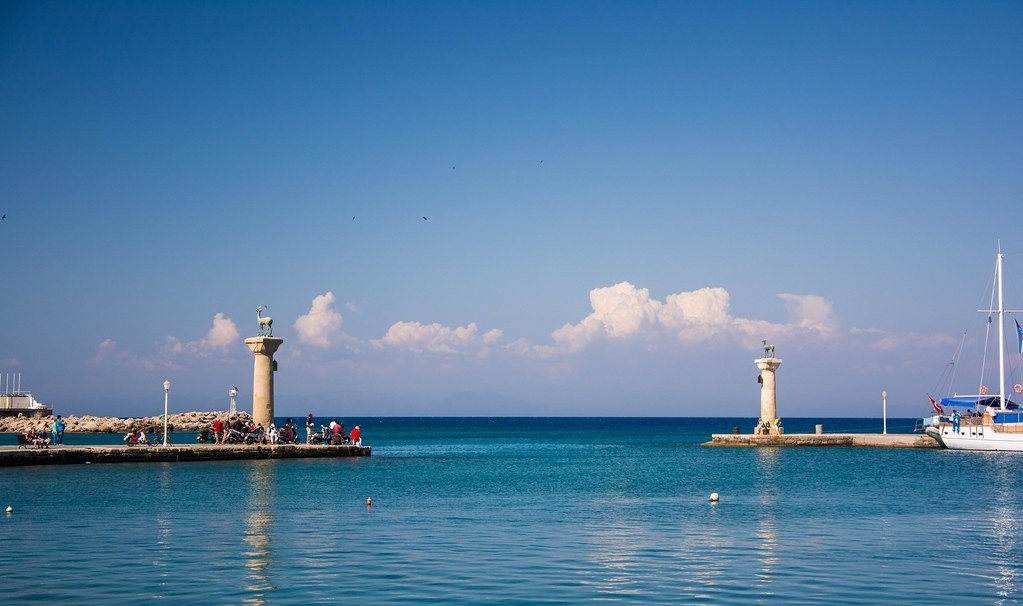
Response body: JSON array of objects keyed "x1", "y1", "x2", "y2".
[
  {"x1": 928, "y1": 395, "x2": 943, "y2": 413},
  {"x1": 1014, "y1": 318, "x2": 1023, "y2": 353}
]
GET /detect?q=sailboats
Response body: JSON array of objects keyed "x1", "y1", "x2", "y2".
[{"x1": 914, "y1": 239, "x2": 1023, "y2": 453}]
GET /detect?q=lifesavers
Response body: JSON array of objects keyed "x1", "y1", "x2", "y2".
[
  {"x1": 1013, "y1": 383, "x2": 1023, "y2": 393},
  {"x1": 980, "y1": 386, "x2": 987, "y2": 395}
]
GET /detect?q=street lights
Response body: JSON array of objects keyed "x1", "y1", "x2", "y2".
[
  {"x1": 882, "y1": 391, "x2": 888, "y2": 433},
  {"x1": 163, "y1": 381, "x2": 170, "y2": 447}
]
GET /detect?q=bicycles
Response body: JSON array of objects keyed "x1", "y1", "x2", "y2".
[{"x1": 150, "y1": 431, "x2": 171, "y2": 447}]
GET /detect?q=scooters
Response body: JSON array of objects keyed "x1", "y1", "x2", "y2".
[
  {"x1": 124, "y1": 428, "x2": 150, "y2": 447},
  {"x1": 228, "y1": 426, "x2": 249, "y2": 444},
  {"x1": 197, "y1": 428, "x2": 216, "y2": 444},
  {"x1": 244, "y1": 422, "x2": 267, "y2": 445},
  {"x1": 276, "y1": 425, "x2": 300, "y2": 445},
  {"x1": 310, "y1": 424, "x2": 332, "y2": 446}
]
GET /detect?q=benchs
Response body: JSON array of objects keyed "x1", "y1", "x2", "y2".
[{"x1": 17, "y1": 435, "x2": 51, "y2": 449}]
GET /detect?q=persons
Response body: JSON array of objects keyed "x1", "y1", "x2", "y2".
[
  {"x1": 26, "y1": 428, "x2": 50, "y2": 449},
  {"x1": 350, "y1": 426, "x2": 362, "y2": 445},
  {"x1": 757, "y1": 418, "x2": 770, "y2": 434},
  {"x1": 214, "y1": 417, "x2": 262, "y2": 444},
  {"x1": 267, "y1": 424, "x2": 278, "y2": 444},
  {"x1": 306, "y1": 414, "x2": 314, "y2": 445},
  {"x1": 774, "y1": 417, "x2": 781, "y2": 435},
  {"x1": 953, "y1": 410, "x2": 961, "y2": 417},
  {"x1": 975, "y1": 410, "x2": 982, "y2": 417},
  {"x1": 330, "y1": 420, "x2": 350, "y2": 445},
  {"x1": 967, "y1": 409, "x2": 974, "y2": 417},
  {"x1": 53, "y1": 415, "x2": 66, "y2": 446},
  {"x1": 285, "y1": 418, "x2": 292, "y2": 444}
]
